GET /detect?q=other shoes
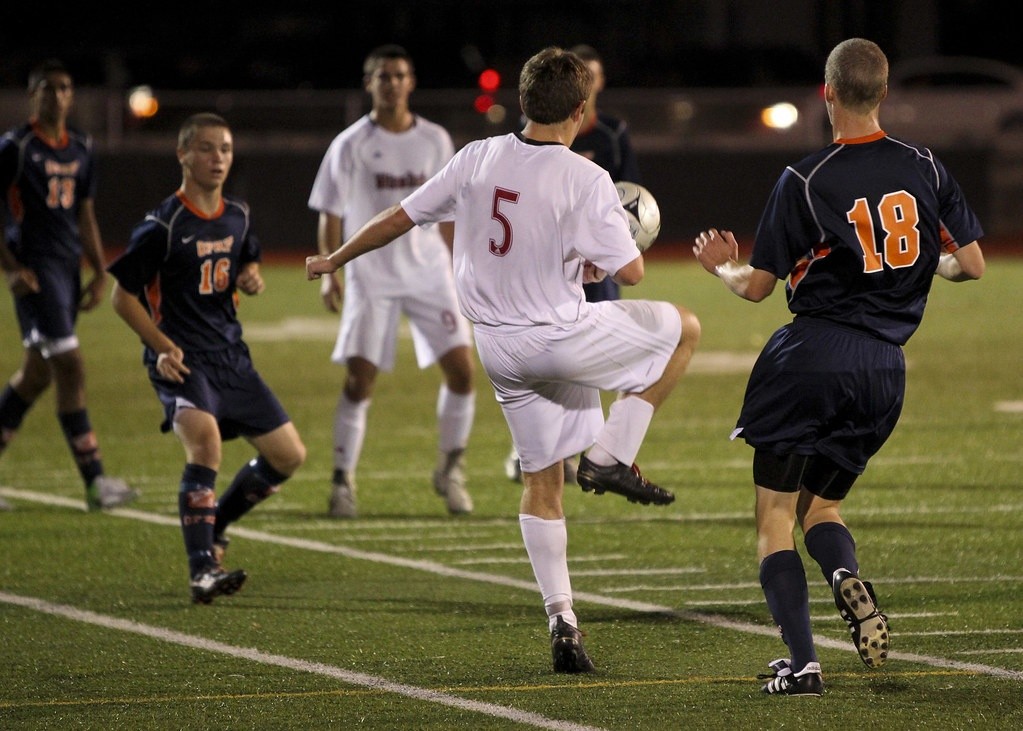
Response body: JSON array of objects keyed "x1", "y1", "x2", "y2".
[{"x1": 505, "y1": 452, "x2": 520, "y2": 481}]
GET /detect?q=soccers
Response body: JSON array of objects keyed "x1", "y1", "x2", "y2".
[{"x1": 615, "y1": 182, "x2": 661, "y2": 254}]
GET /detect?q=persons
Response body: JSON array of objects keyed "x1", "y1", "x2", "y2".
[
  {"x1": 106, "y1": 114, "x2": 306, "y2": 608},
  {"x1": 694, "y1": 37, "x2": 984, "y2": 698},
  {"x1": 0, "y1": 63, "x2": 139, "y2": 515},
  {"x1": 303, "y1": 49, "x2": 701, "y2": 673},
  {"x1": 308, "y1": 50, "x2": 478, "y2": 517},
  {"x1": 504, "y1": 47, "x2": 635, "y2": 483}
]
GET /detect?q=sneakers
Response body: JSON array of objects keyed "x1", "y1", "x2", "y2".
[
  {"x1": 435, "y1": 470, "x2": 472, "y2": 512},
  {"x1": 758, "y1": 658, "x2": 825, "y2": 697},
  {"x1": 577, "y1": 451, "x2": 674, "y2": 505},
  {"x1": 834, "y1": 572, "x2": 889, "y2": 669},
  {"x1": 550, "y1": 615, "x2": 594, "y2": 673},
  {"x1": 87, "y1": 478, "x2": 133, "y2": 509},
  {"x1": 190, "y1": 562, "x2": 246, "y2": 604},
  {"x1": 213, "y1": 537, "x2": 228, "y2": 565},
  {"x1": 329, "y1": 487, "x2": 356, "y2": 517}
]
